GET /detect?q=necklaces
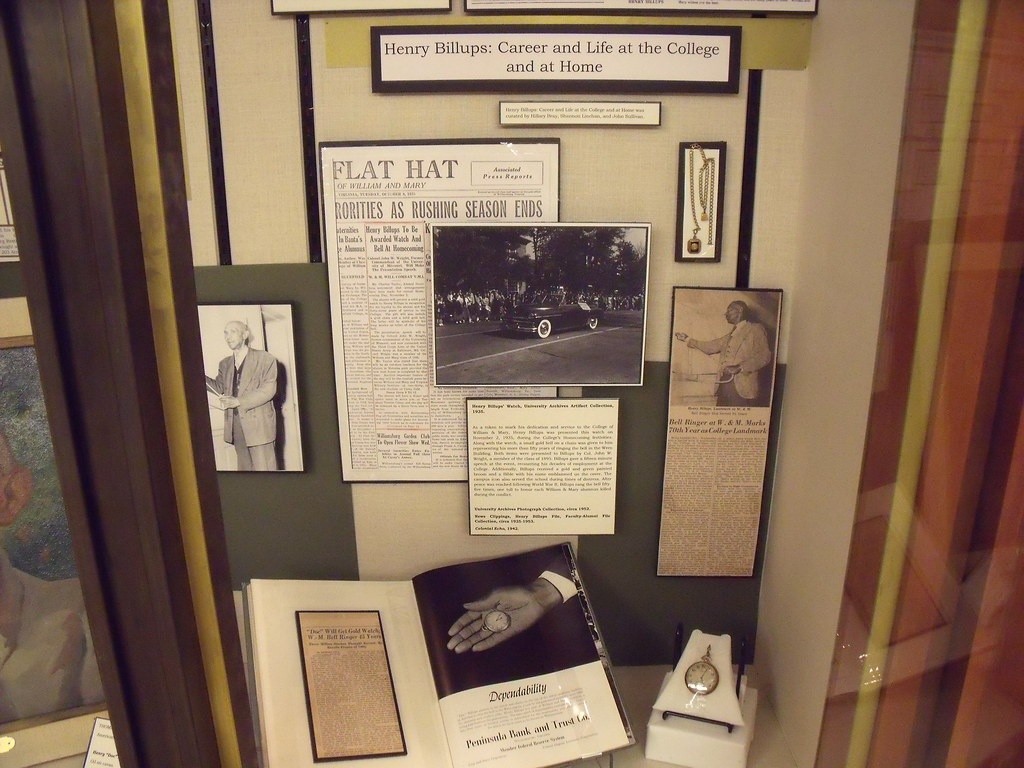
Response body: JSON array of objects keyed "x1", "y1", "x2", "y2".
[{"x1": 687, "y1": 143, "x2": 715, "y2": 256}]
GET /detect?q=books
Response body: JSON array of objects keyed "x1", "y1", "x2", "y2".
[{"x1": 240, "y1": 541, "x2": 635, "y2": 768}]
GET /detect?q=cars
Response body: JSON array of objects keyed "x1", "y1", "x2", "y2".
[{"x1": 503, "y1": 294, "x2": 604, "y2": 339}]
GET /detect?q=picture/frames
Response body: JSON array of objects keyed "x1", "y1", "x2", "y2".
[
  {"x1": 198, "y1": 305, "x2": 305, "y2": 472},
  {"x1": 0, "y1": 336, "x2": 108, "y2": 738},
  {"x1": 432, "y1": 223, "x2": 651, "y2": 388}
]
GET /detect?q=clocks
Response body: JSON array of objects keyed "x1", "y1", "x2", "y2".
[
  {"x1": 684, "y1": 644, "x2": 719, "y2": 695},
  {"x1": 482, "y1": 610, "x2": 512, "y2": 633}
]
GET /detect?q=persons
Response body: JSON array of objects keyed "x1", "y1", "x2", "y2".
[
  {"x1": 204, "y1": 320, "x2": 278, "y2": 470},
  {"x1": 675, "y1": 300, "x2": 771, "y2": 407},
  {"x1": 434, "y1": 288, "x2": 644, "y2": 326},
  {"x1": 446, "y1": 553, "x2": 578, "y2": 656}
]
